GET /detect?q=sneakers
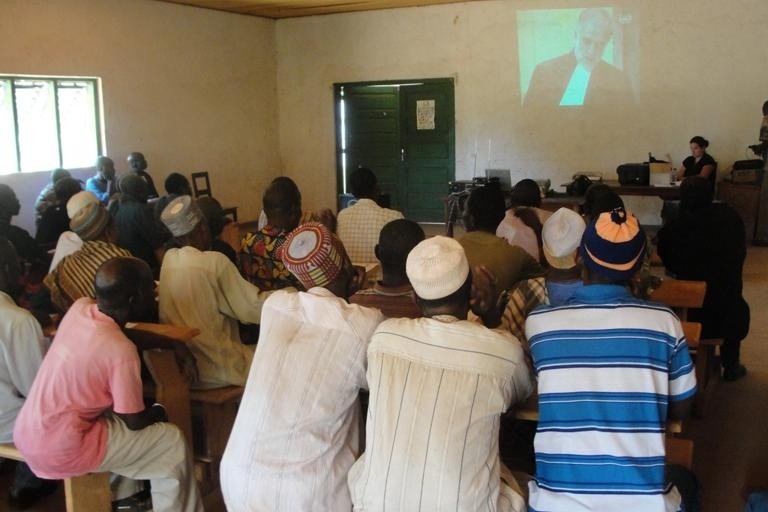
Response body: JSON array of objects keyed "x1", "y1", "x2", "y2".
[{"x1": 111, "y1": 489, "x2": 152, "y2": 512}]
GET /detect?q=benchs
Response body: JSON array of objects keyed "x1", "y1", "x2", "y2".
[{"x1": 0, "y1": 443, "x2": 112, "y2": 512}]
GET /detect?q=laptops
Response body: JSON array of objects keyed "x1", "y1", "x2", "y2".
[{"x1": 485, "y1": 169, "x2": 513, "y2": 192}]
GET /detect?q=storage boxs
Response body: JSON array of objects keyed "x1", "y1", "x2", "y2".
[{"x1": 731, "y1": 160, "x2": 764, "y2": 183}]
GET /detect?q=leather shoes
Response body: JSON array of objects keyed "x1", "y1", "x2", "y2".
[
  {"x1": 723, "y1": 363, "x2": 746, "y2": 381},
  {"x1": 8, "y1": 485, "x2": 55, "y2": 505}
]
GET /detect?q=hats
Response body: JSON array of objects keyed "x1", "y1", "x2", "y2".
[
  {"x1": 542, "y1": 207, "x2": 648, "y2": 279},
  {"x1": 406, "y1": 236, "x2": 470, "y2": 301},
  {"x1": 66, "y1": 192, "x2": 111, "y2": 241},
  {"x1": 282, "y1": 221, "x2": 344, "y2": 289},
  {"x1": 160, "y1": 195, "x2": 203, "y2": 236}
]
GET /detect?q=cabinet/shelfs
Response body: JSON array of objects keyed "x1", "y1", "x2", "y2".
[{"x1": 719, "y1": 180, "x2": 760, "y2": 242}]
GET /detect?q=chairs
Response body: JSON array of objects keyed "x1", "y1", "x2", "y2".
[
  {"x1": 649, "y1": 280, "x2": 724, "y2": 419},
  {"x1": 192, "y1": 172, "x2": 238, "y2": 222},
  {"x1": 710, "y1": 162, "x2": 717, "y2": 192},
  {"x1": 125, "y1": 322, "x2": 244, "y2": 496}
]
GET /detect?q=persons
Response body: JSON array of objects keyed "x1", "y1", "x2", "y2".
[
  {"x1": 15, "y1": 152, "x2": 305, "y2": 512},
  {"x1": 456, "y1": 174, "x2": 751, "y2": 511},
  {"x1": 521, "y1": 8, "x2": 636, "y2": 109},
  {"x1": 0, "y1": 183, "x2": 66, "y2": 512},
  {"x1": 221, "y1": 168, "x2": 535, "y2": 512},
  {"x1": 677, "y1": 137, "x2": 715, "y2": 186}
]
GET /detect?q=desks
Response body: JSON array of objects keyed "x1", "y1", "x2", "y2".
[
  {"x1": 516, "y1": 385, "x2": 686, "y2": 433},
  {"x1": 351, "y1": 262, "x2": 380, "y2": 276},
  {"x1": 561, "y1": 180, "x2": 679, "y2": 195}
]
